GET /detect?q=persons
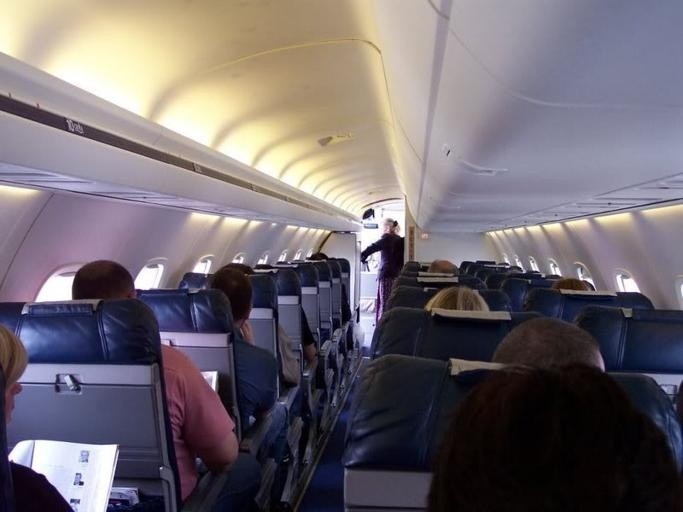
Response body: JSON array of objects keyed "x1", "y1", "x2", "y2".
[
  {"x1": 73, "y1": 473, "x2": 84, "y2": 486},
  {"x1": 552, "y1": 276, "x2": 592, "y2": 291},
  {"x1": 277, "y1": 252, "x2": 352, "y2": 323},
  {"x1": 254, "y1": 263, "x2": 315, "y2": 363},
  {"x1": 205, "y1": 267, "x2": 291, "y2": 464},
  {"x1": 423, "y1": 361, "x2": 680, "y2": 511},
  {"x1": 492, "y1": 315, "x2": 608, "y2": 373},
  {"x1": 361, "y1": 217, "x2": 405, "y2": 326},
  {"x1": 427, "y1": 259, "x2": 460, "y2": 274},
  {"x1": 425, "y1": 284, "x2": 488, "y2": 312},
  {"x1": 72, "y1": 259, "x2": 264, "y2": 512},
  {"x1": 81, "y1": 451, "x2": 89, "y2": 463},
  {"x1": 222, "y1": 261, "x2": 303, "y2": 416},
  {"x1": 0, "y1": 324, "x2": 76, "y2": 512}
]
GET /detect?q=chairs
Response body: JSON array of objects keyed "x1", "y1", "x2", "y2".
[{"x1": 0, "y1": 256, "x2": 683, "y2": 512}]
